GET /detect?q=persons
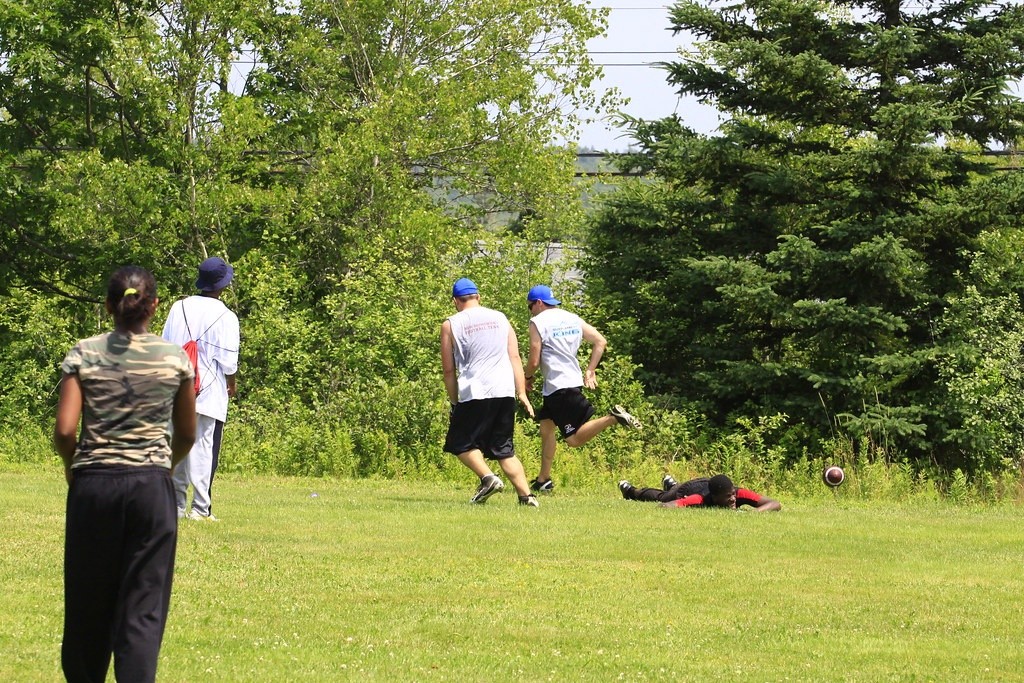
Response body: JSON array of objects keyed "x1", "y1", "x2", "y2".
[
  {"x1": 54, "y1": 265, "x2": 196, "y2": 683},
  {"x1": 524, "y1": 285, "x2": 643, "y2": 494},
  {"x1": 618, "y1": 473, "x2": 782, "y2": 513},
  {"x1": 156, "y1": 258, "x2": 241, "y2": 518},
  {"x1": 439, "y1": 278, "x2": 540, "y2": 506}
]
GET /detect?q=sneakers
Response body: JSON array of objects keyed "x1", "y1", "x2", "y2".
[
  {"x1": 470, "y1": 474, "x2": 504, "y2": 504},
  {"x1": 662, "y1": 475, "x2": 676, "y2": 491},
  {"x1": 618, "y1": 480, "x2": 632, "y2": 501},
  {"x1": 187, "y1": 509, "x2": 220, "y2": 521},
  {"x1": 610, "y1": 405, "x2": 643, "y2": 430},
  {"x1": 530, "y1": 476, "x2": 554, "y2": 494},
  {"x1": 178, "y1": 510, "x2": 190, "y2": 519},
  {"x1": 518, "y1": 494, "x2": 539, "y2": 507}
]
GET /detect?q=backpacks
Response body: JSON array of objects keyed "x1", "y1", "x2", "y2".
[{"x1": 182, "y1": 340, "x2": 200, "y2": 399}]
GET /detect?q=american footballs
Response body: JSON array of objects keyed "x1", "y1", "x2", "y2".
[{"x1": 822, "y1": 466, "x2": 846, "y2": 488}]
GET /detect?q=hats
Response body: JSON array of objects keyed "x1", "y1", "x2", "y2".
[
  {"x1": 452, "y1": 277, "x2": 478, "y2": 297},
  {"x1": 195, "y1": 257, "x2": 234, "y2": 291},
  {"x1": 528, "y1": 285, "x2": 562, "y2": 306}
]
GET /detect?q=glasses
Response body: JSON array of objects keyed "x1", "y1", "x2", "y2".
[{"x1": 528, "y1": 301, "x2": 537, "y2": 310}]
